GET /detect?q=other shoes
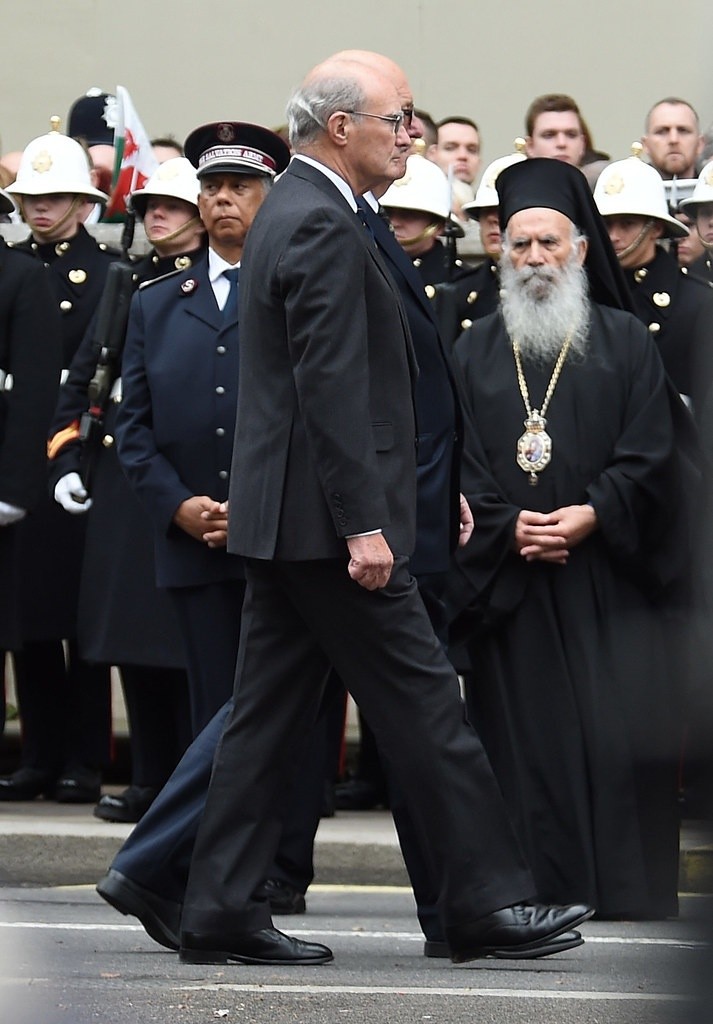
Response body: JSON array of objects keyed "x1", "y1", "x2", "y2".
[
  {"x1": 94, "y1": 785, "x2": 154, "y2": 822},
  {"x1": 332, "y1": 776, "x2": 378, "y2": 812},
  {"x1": 264, "y1": 879, "x2": 307, "y2": 915},
  {"x1": 43, "y1": 776, "x2": 101, "y2": 805},
  {"x1": 1, "y1": 766, "x2": 53, "y2": 802}
]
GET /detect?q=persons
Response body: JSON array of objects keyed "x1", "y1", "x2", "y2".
[{"x1": 0, "y1": 39, "x2": 713, "y2": 969}]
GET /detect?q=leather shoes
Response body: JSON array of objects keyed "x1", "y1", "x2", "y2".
[
  {"x1": 95, "y1": 867, "x2": 184, "y2": 949},
  {"x1": 451, "y1": 900, "x2": 596, "y2": 962},
  {"x1": 181, "y1": 920, "x2": 334, "y2": 967},
  {"x1": 424, "y1": 920, "x2": 585, "y2": 960}
]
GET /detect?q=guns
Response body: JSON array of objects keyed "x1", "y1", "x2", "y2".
[{"x1": 74, "y1": 149, "x2": 142, "y2": 504}]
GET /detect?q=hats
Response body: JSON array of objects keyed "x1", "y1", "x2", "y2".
[
  {"x1": 65, "y1": 93, "x2": 125, "y2": 145},
  {"x1": 184, "y1": 121, "x2": 293, "y2": 178},
  {"x1": 494, "y1": 157, "x2": 589, "y2": 235},
  {"x1": 662, "y1": 177, "x2": 700, "y2": 211}
]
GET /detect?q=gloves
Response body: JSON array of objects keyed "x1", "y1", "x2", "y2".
[{"x1": 53, "y1": 472, "x2": 93, "y2": 514}]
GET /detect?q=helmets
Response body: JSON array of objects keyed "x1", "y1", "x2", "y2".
[
  {"x1": 594, "y1": 156, "x2": 690, "y2": 239},
  {"x1": 462, "y1": 153, "x2": 531, "y2": 223},
  {"x1": 4, "y1": 131, "x2": 110, "y2": 203},
  {"x1": 127, "y1": 157, "x2": 202, "y2": 212},
  {"x1": 377, "y1": 154, "x2": 467, "y2": 239},
  {"x1": 677, "y1": 160, "x2": 713, "y2": 218}
]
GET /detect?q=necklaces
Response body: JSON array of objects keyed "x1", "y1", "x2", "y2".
[{"x1": 508, "y1": 316, "x2": 571, "y2": 485}]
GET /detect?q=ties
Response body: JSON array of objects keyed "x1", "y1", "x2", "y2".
[{"x1": 220, "y1": 268, "x2": 242, "y2": 325}]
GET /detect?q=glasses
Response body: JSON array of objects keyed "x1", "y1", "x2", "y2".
[
  {"x1": 401, "y1": 107, "x2": 416, "y2": 131},
  {"x1": 343, "y1": 110, "x2": 404, "y2": 133}
]
